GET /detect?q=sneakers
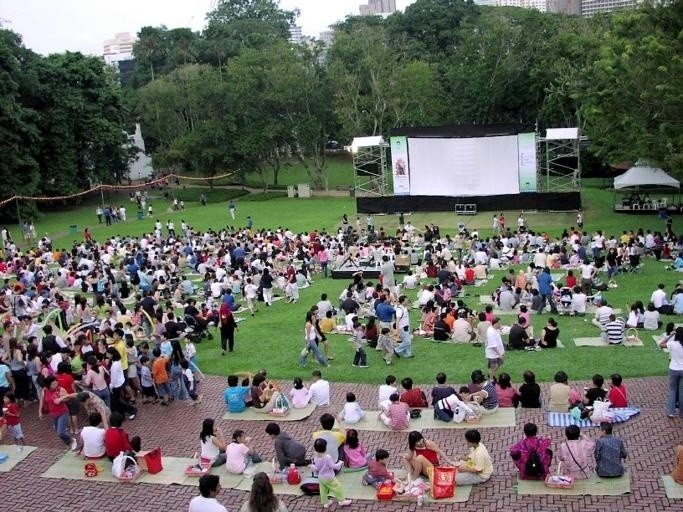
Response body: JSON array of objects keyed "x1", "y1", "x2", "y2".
[
  {"x1": 169, "y1": 397, "x2": 174, "y2": 402},
  {"x1": 143, "y1": 400, "x2": 151, "y2": 405},
  {"x1": 360, "y1": 365, "x2": 369, "y2": 368},
  {"x1": 161, "y1": 400, "x2": 170, "y2": 406},
  {"x1": 70, "y1": 437, "x2": 78, "y2": 451},
  {"x1": 152, "y1": 399, "x2": 160, "y2": 404},
  {"x1": 352, "y1": 364, "x2": 359, "y2": 367}
]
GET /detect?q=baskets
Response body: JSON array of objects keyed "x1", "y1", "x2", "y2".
[
  {"x1": 185, "y1": 452, "x2": 212, "y2": 477},
  {"x1": 625, "y1": 327, "x2": 639, "y2": 344},
  {"x1": 269, "y1": 396, "x2": 289, "y2": 416},
  {"x1": 464, "y1": 401, "x2": 482, "y2": 424},
  {"x1": 117, "y1": 456, "x2": 144, "y2": 482},
  {"x1": 590, "y1": 405, "x2": 613, "y2": 423},
  {"x1": 544, "y1": 461, "x2": 575, "y2": 489}
]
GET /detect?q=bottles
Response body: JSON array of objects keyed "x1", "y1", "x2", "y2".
[{"x1": 416, "y1": 493, "x2": 423, "y2": 506}]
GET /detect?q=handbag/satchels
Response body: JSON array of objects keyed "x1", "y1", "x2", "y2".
[
  {"x1": 143, "y1": 447, "x2": 163, "y2": 474},
  {"x1": 377, "y1": 477, "x2": 396, "y2": 500},
  {"x1": 425, "y1": 466, "x2": 457, "y2": 499},
  {"x1": 592, "y1": 397, "x2": 613, "y2": 420}
]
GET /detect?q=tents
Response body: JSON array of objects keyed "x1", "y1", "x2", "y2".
[{"x1": 613, "y1": 155, "x2": 680, "y2": 214}]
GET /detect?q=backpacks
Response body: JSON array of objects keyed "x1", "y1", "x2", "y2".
[{"x1": 522, "y1": 439, "x2": 545, "y2": 479}]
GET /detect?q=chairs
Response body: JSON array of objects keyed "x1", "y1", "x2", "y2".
[
  {"x1": 661, "y1": 198, "x2": 667, "y2": 208},
  {"x1": 643, "y1": 200, "x2": 650, "y2": 209},
  {"x1": 632, "y1": 200, "x2": 639, "y2": 210}
]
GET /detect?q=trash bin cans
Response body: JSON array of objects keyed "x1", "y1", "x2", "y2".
[
  {"x1": 348, "y1": 186, "x2": 355, "y2": 196},
  {"x1": 315, "y1": 183, "x2": 322, "y2": 191}
]
